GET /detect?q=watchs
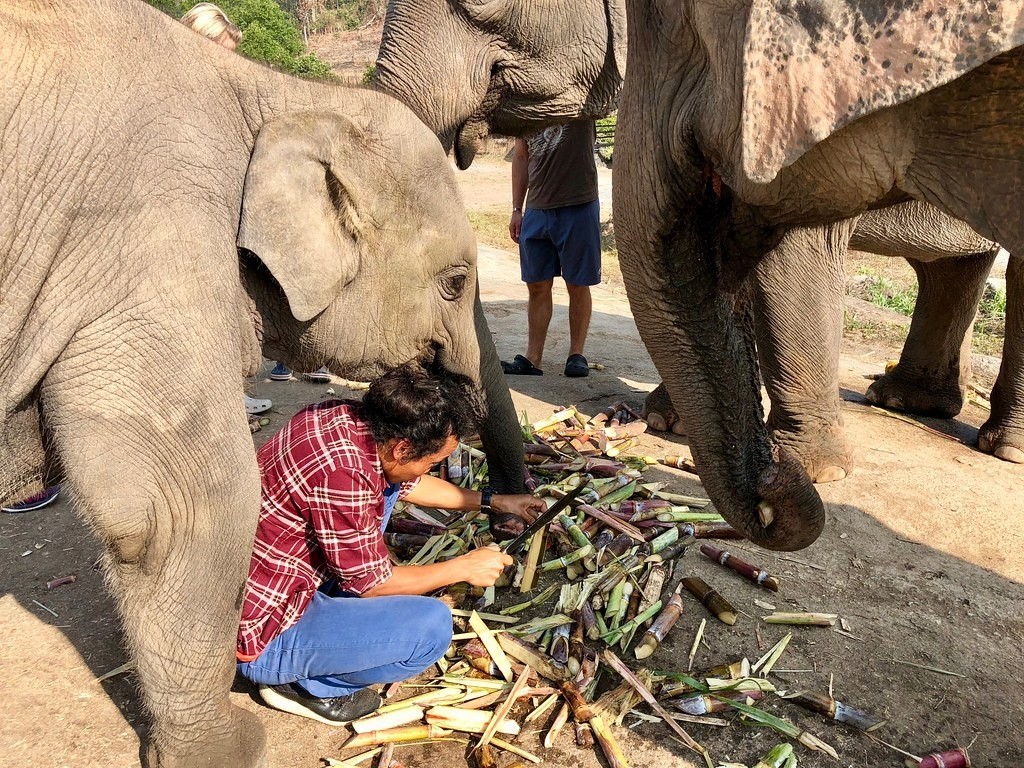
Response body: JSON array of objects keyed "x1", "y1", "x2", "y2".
[{"x1": 480, "y1": 486, "x2": 498, "y2": 514}]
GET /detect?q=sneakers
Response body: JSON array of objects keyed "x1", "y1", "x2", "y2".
[{"x1": 258, "y1": 681, "x2": 384, "y2": 726}]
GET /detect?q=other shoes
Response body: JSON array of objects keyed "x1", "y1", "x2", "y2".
[
  {"x1": 244, "y1": 394, "x2": 272, "y2": 413},
  {"x1": 271, "y1": 364, "x2": 293, "y2": 379},
  {"x1": 1, "y1": 486, "x2": 60, "y2": 512},
  {"x1": 303, "y1": 367, "x2": 331, "y2": 384}
]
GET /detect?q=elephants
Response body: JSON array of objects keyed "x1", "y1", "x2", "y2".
[{"x1": 0, "y1": 0, "x2": 1024, "y2": 768}]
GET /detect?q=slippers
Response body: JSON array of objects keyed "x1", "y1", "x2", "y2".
[
  {"x1": 500, "y1": 354, "x2": 543, "y2": 375},
  {"x1": 564, "y1": 353, "x2": 589, "y2": 377}
]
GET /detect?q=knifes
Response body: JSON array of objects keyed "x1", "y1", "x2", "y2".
[{"x1": 499, "y1": 480, "x2": 589, "y2": 554}]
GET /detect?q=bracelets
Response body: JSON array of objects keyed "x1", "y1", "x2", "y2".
[{"x1": 513, "y1": 207, "x2": 521, "y2": 211}]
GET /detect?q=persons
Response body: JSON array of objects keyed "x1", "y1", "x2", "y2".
[
  {"x1": 1, "y1": 475, "x2": 68, "y2": 512},
  {"x1": 237, "y1": 364, "x2": 547, "y2": 726},
  {"x1": 500, "y1": 119, "x2": 602, "y2": 377},
  {"x1": 180, "y1": 1, "x2": 272, "y2": 414},
  {"x1": 271, "y1": 363, "x2": 332, "y2": 380}
]
